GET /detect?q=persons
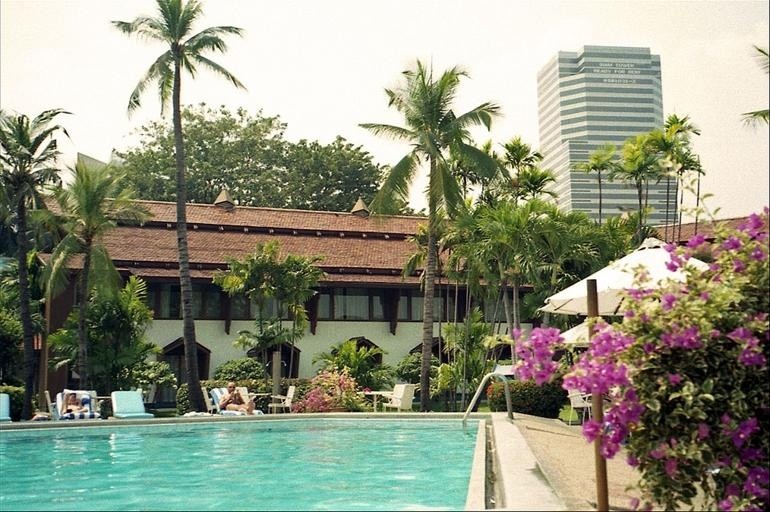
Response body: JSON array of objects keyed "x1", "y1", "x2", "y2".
[
  {"x1": 218, "y1": 382, "x2": 255, "y2": 414},
  {"x1": 61, "y1": 392, "x2": 88, "y2": 413}
]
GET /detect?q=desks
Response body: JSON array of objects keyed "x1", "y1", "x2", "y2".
[
  {"x1": 357, "y1": 390, "x2": 393, "y2": 412},
  {"x1": 242, "y1": 392, "x2": 271, "y2": 409}
]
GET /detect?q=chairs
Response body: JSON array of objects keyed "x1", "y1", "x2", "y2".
[
  {"x1": 383, "y1": 384, "x2": 416, "y2": 412},
  {"x1": 0, "y1": 393, "x2": 12, "y2": 421},
  {"x1": 209, "y1": 387, "x2": 264, "y2": 415},
  {"x1": 383, "y1": 385, "x2": 406, "y2": 411},
  {"x1": 202, "y1": 387, "x2": 218, "y2": 415},
  {"x1": 234, "y1": 387, "x2": 250, "y2": 405},
  {"x1": 31, "y1": 389, "x2": 154, "y2": 422},
  {"x1": 566, "y1": 386, "x2": 593, "y2": 427},
  {"x1": 268, "y1": 386, "x2": 295, "y2": 414}
]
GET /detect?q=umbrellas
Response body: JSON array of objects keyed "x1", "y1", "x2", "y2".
[{"x1": 536, "y1": 237, "x2": 714, "y2": 348}]
